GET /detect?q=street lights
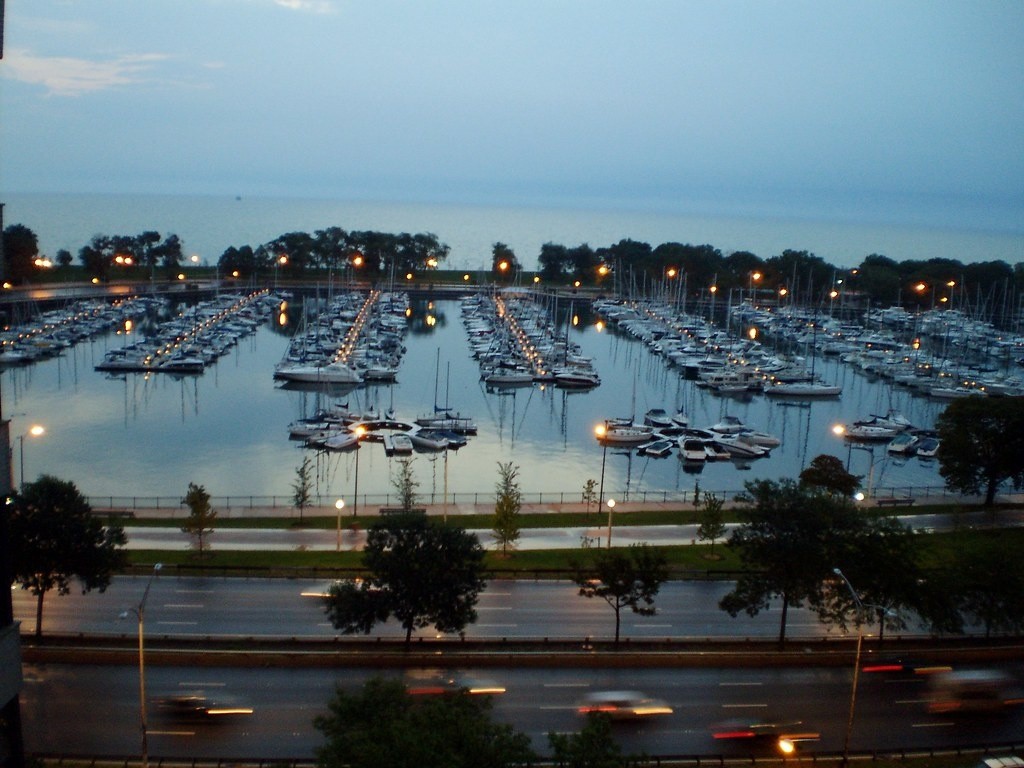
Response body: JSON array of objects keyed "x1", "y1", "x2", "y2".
[
  {"x1": 834, "y1": 568, "x2": 899, "y2": 768},
  {"x1": 19, "y1": 425, "x2": 44, "y2": 494},
  {"x1": 119, "y1": 562, "x2": 163, "y2": 768},
  {"x1": 834, "y1": 425, "x2": 854, "y2": 509},
  {"x1": 608, "y1": 501, "x2": 616, "y2": 557},
  {"x1": 354, "y1": 427, "x2": 366, "y2": 526},
  {"x1": 336, "y1": 500, "x2": 345, "y2": 550},
  {"x1": 597, "y1": 426, "x2": 608, "y2": 516}
]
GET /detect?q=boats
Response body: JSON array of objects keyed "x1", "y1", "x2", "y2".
[
  {"x1": 846, "y1": 409, "x2": 939, "y2": 459},
  {"x1": 599, "y1": 407, "x2": 782, "y2": 463},
  {"x1": 288, "y1": 399, "x2": 478, "y2": 453}
]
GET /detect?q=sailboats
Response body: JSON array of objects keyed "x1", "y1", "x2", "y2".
[
  {"x1": 0, "y1": 257, "x2": 409, "y2": 382},
  {"x1": 459, "y1": 261, "x2": 1023, "y2": 399}
]
